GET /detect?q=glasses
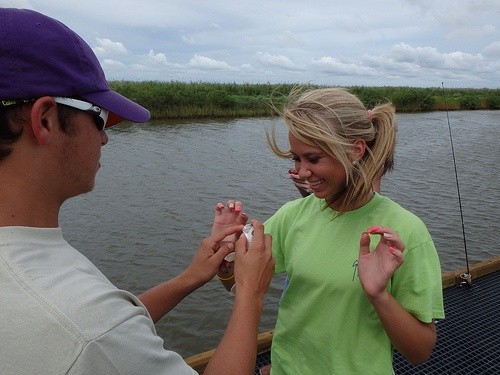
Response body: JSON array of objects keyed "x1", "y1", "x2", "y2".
[{"x1": 2, "y1": 97, "x2": 109, "y2": 131}]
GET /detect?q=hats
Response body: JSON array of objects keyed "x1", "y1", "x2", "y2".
[{"x1": 0, "y1": 8, "x2": 151, "y2": 123}]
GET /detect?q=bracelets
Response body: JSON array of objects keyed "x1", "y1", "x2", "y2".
[{"x1": 215, "y1": 274, "x2": 235, "y2": 280}]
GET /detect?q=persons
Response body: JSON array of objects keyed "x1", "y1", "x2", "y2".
[
  {"x1": 0, "y1": 7, "x2": 277, "y2": 375},
  {"x1": 211, "y1": 87, "x2": 445, "y2": 375}
]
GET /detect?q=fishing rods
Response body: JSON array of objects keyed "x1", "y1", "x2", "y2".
[{"x1": 442, "y1": 82, "x2": 472, "y2": 287}]
